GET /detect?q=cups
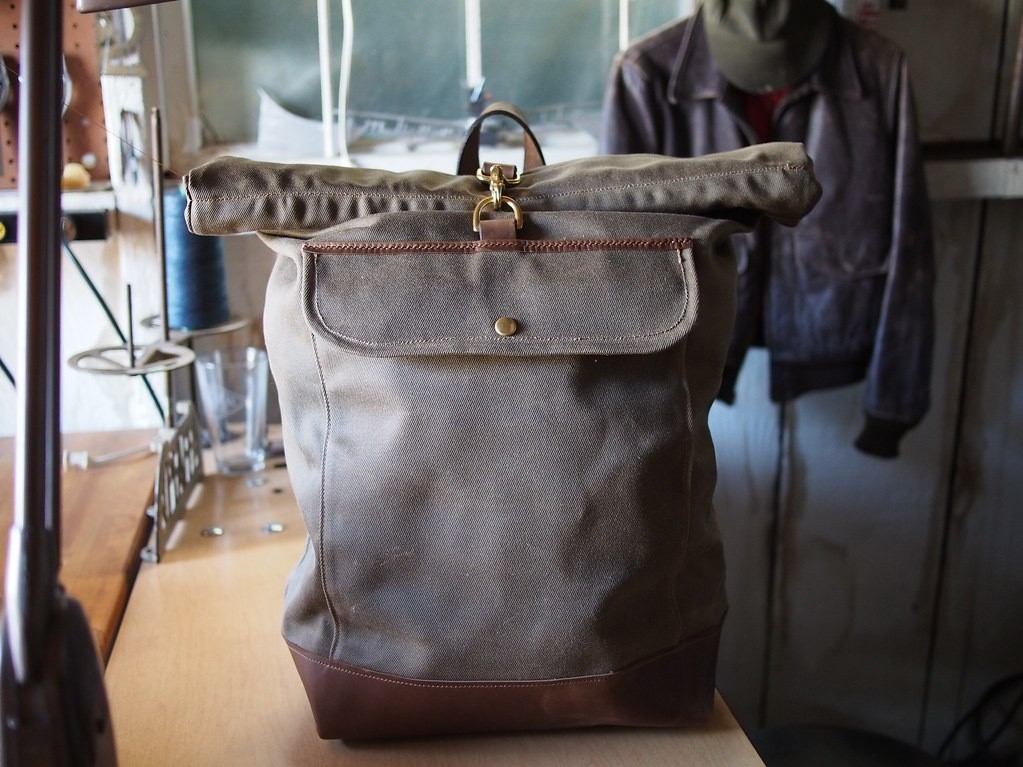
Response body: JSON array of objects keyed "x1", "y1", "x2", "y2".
[{"x1": 192, "y1": 346, "x2": 274, "y2": 476}]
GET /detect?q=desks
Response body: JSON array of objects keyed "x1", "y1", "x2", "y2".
[{"x1": 104, "y1": 467, "x2": 766, "y2": 767}]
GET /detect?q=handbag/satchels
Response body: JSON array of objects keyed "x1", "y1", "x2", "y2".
[{"x1": 179, "y1": 103, "x2": 821, "y2": 742}]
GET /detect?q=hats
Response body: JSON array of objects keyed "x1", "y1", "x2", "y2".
[{"x1": 704, "y1": 0, "x2": 833, "y2": 94}]
